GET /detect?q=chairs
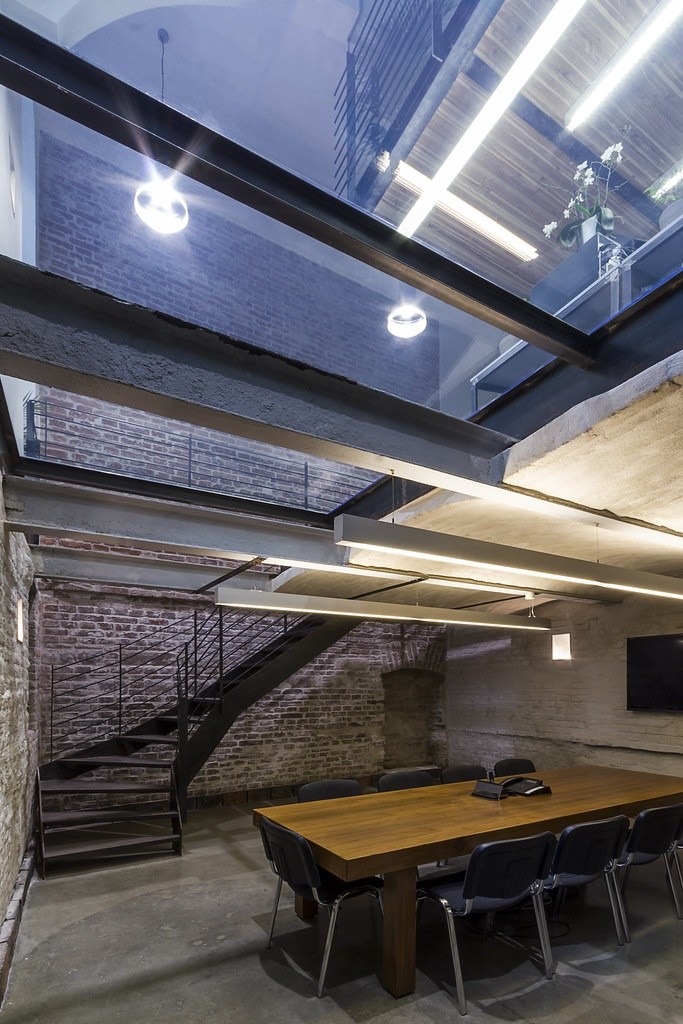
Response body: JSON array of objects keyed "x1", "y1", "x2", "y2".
[
  {"x1": 415, "y1": 802, "x2": 683, "y2": 1018},
  {"x1": 297, "y1": 759, "x2": 537, "y2": 879},
  {"x1": 259, "y1": 816, "x2": 385, "y2": 999}
]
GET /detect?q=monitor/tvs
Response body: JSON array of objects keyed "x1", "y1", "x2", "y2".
[{"x1": 627, "y1": 633, "x2": 683, "y2": 712}]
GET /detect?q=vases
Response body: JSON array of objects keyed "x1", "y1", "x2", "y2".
[
  {"x1": 577, "y1": 215, "x2": 598, "y2": 244},
  {"x1": 658, "y1": 198, "x2": 683, "y2": 230}
]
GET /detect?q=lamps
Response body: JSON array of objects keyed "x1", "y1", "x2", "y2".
[
  {"x1": 134, "y1": 179, "x2": 190, "y2": 233},
  {"x1": 334, "y1": 513, "x2": 683, "y2": 600},
  {"x1": 215, "y1": 586, "x2": 551, "y2": 631},
  {"x1": 387, "y1": 305, "x2": 427, "y2": 338}
]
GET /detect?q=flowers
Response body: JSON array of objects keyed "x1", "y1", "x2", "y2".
[
  {"x1": 647, "y1": 162, "x2": 683, "y2": 205},
  {"x1": 536, "y1": 141, "x2": 633, "y2": 248}
]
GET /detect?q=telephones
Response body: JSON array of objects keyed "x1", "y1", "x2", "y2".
[{"x1": 499, "y1": 776, "x2": 552, "y2": 796}]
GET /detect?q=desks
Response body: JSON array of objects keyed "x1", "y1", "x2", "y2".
[
  {"x1": 253, "y1": 765, "x2": 683, "y2": 998},
  {"x1": 470, "y1": 215, "x2": 683, "y2": 413}
]
